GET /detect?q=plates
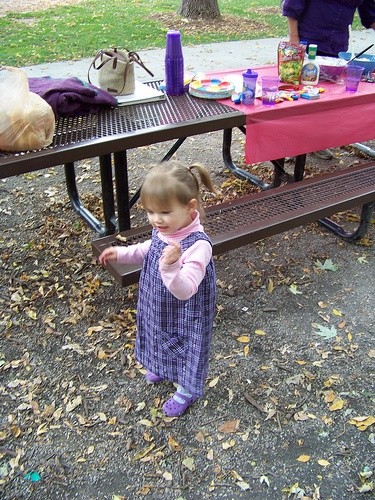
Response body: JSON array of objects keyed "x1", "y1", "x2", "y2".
[{"x1": 161, "y1": 71, "x2": 234, "y2": 100}]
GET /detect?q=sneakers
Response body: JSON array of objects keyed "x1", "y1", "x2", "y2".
[
  {"x1": 313, "y1": 149, "x2": 333, "y2": 159},
  {"x1": 285, "y1": 156, "x2": 293, "y2": 162}
]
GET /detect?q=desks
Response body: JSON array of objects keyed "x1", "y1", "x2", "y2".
[{"x1": 0, "y1": 62, "x2": 375, "y2": 247}]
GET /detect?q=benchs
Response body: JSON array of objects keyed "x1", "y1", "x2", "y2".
[{"x1": 91, "y1": 160, "x2": 375, "y2": 288}]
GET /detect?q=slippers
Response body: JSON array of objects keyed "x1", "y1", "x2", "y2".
[
  {"x1": 163, "y1": 391, "x2": 196, "y2": 417},
  {"x1": 144, "y1": 370, "x2": 163, "y2": 381}
]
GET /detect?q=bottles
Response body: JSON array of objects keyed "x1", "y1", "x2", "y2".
[
  {"x1": 300, "y1": 44, "x2": 320, "y2": 85},
  {"x1": 299, "y1": 41, "x2": 308, "y2": 66},
  {"x1": 242, "y1": 69, "x2": 258, "y2": 104}
]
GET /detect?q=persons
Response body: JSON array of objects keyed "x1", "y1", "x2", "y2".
[
  {"x1": 282, "y1": 0, "x2": 375, "y2": 162},
  {"x1": 99, "y1": 163, "x2": 217, "y2": 416}
]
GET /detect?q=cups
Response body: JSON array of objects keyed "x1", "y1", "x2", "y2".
[
  {"x1": 261, "y1": 76, "x2": 280, "y2": 105},
  {"x1": 345, "y1": 64, "x2": 365, "y2": 92},
  {"x1": 165, "y1": 32, "x2": 184, "y2": 96}
]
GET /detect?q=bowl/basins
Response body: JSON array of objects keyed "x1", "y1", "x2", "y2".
[
  {"x1": 338, "y1": 52, "x2": 375, "y2": 74},
  {"x1": 317, "y1": 55, "x2": 347, "y2": 78}
]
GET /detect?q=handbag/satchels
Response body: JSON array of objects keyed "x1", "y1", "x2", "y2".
[
  {"x1": 0, "y1": 65, "x2": 56, "y2": 152},
  {"x1": 88, "y1": 47, "x2": 154, "y2": 97}
]
